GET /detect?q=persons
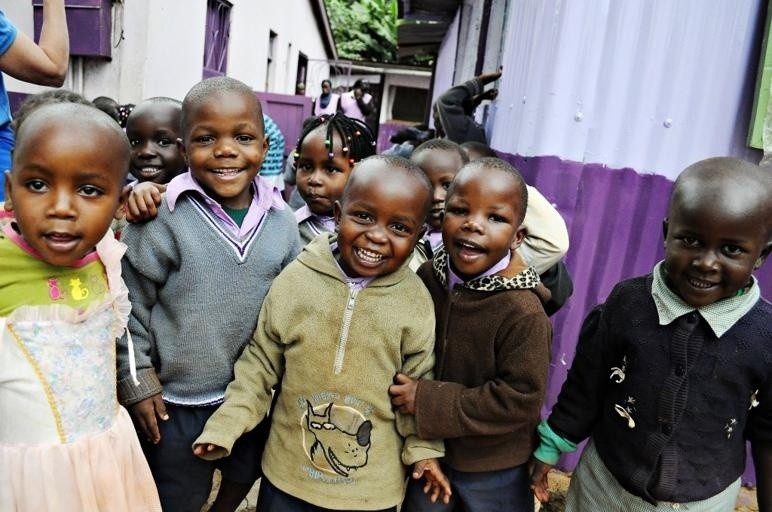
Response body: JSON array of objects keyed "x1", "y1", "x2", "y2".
[
  {"x1": 0, "y1": 0, "x2": 71, "y2": 203},
  {"x1": 193, "y1": 156, "x2": 452, "y2": 512},
  {"x1": 0, "y1": 102, "x2": 162, "y2": 511},
  {"x1": 312, "y1": 79, "x2": 374, "y2": 128},
  {"x1": 387, "y1": 156, "x2": 553, "y2": 512},
  {"x1": 116, "y1": 76, "x2": 300, "y2": 512},
  {"x1": 530, "y1": 157, "x2": 771, "y2": 511},
  {"x1": 12, "y1": 92, "x2": 573, "y2": 316},
  {"x1": 432, "y1": 67, "x2": 503, "y2": 144}
]
